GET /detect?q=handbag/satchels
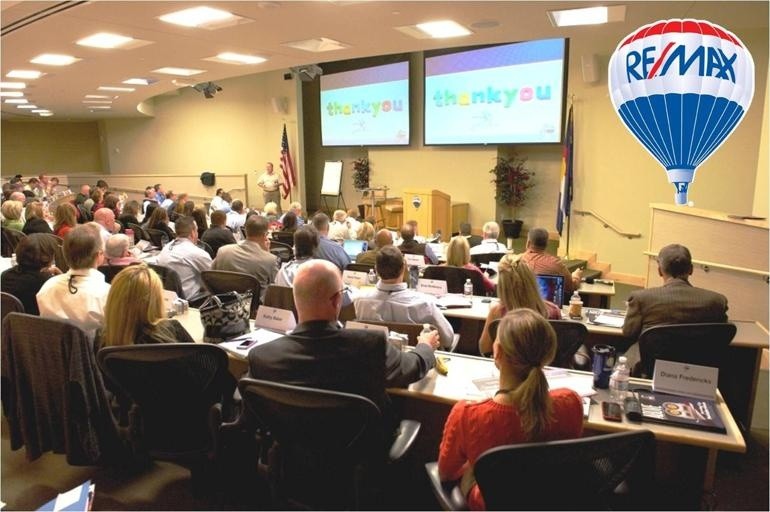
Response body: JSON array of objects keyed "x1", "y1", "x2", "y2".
[{"x1": 199, "y1": 289, "x2": 254, "y2": 344}]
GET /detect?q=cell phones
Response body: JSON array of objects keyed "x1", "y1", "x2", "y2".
[
  {"x1": 602, "y1": 400, "x2": 622, "y2": 421},
  {"x1": 237, "y1": 339, "x2": 258, "y2": 349}
]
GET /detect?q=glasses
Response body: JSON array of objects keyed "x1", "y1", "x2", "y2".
[{"x1": 329, "y1": 286, "x2": 348, "y2": 300}]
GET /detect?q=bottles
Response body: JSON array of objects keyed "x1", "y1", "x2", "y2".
[
  {"x1": 368, "y1": 268, "x2": 375, "y2": 283},
  {"x1": 570, "y1": 291, "x2": 582, "y2": 301},
  {"x1": 419, "y1": 322, "x2": 435, "y2": 377},
  {"x1": 610, "y1": 356, "x2": 631, "y2": 403},
  {"x1": 11, "y1": 253, "x2": 17, "y2": 265},
  {"x1": 463, "y1": 278, "x2": 473, "y2": 301},
  {"x1": 569, "y1": 301, "x2": 584, "y2": 319}
]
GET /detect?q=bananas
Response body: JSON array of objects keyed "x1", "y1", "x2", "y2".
[{"x1": 435, "y1": 355, "x2": 451, "y2": 375}]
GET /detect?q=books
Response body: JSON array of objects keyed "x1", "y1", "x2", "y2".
[{"x1": 638, "y1": 391, "x2": 727, "y2": 433}]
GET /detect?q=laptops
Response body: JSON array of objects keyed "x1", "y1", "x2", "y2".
[
  {"x1": 534, "y1": 274, "x2": 565, "y2": 311},
  {"x1": 342, "y1": 239, "x2": 369, "y2": 262}
]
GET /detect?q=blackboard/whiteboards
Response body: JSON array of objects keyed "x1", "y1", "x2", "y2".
[{"x1": 320, "y1": 160, "x2": 343, "y2": 196}]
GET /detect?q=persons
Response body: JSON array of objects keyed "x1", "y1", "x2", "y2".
[
  {"x1": 620, "y1": 245, "x2": 729, "y2": 377},
  {"x1": 0, "y1": 163, "x2": 585, "y2": 512}
]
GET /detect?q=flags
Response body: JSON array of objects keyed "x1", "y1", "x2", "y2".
[
  {"x1": 556, "y1": 105, "x2": 574, "y2": 237},
  {"x1": 276, "y1": 124, "x2": 297, "y2": 199}
]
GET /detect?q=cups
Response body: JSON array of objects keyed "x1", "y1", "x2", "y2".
[
  {"x1": 126, "y1": 229, "x2": 135, "y2": 247},
  {"x1": 591, "y1": 344, "x2": 617, "y2": 389},
  {"x1": 409, "y1": 265, "x2": 419, "y2": 289},
  {"x1": 161, "y1": 235, "x2": 169, "y2": 249}
]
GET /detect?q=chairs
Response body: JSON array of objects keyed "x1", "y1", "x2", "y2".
[{"x1": 0, "y1": 213, "x2": 768, "y2": 512}]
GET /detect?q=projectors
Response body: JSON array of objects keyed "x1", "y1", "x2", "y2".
[
  {"x1": 288, "y1": 64, "x2": 324, "y2": 83},
  {"x1": 193, "y1": 81, "x2": 223, "y2": 99}
]
